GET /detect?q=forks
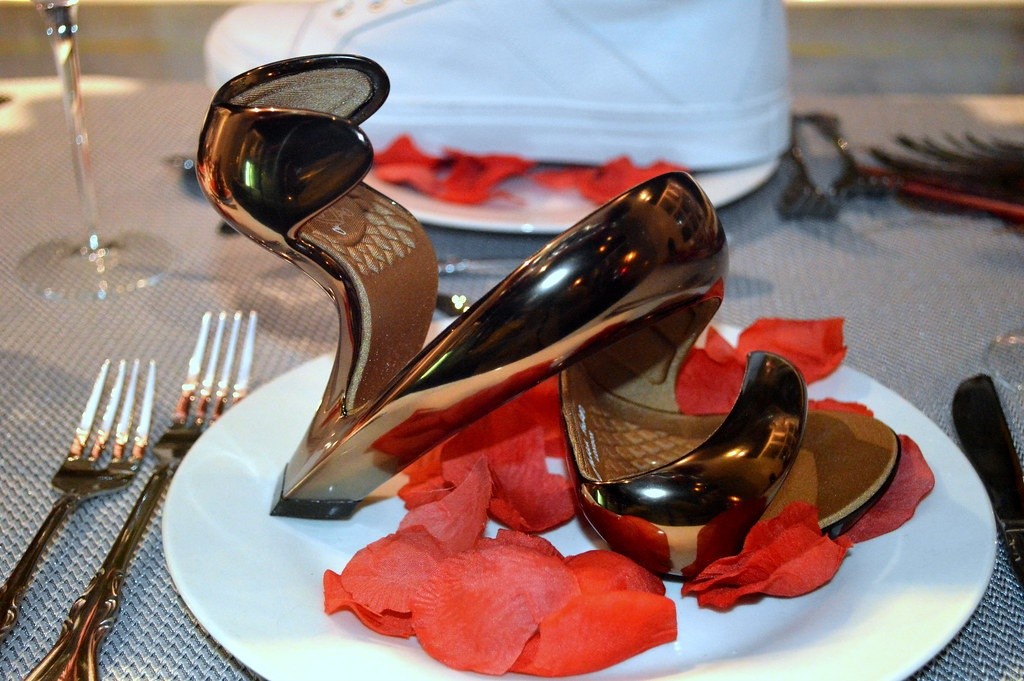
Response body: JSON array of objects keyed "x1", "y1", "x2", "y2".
[
  {"x1": 778, "y1": 114, "x2": 844, "y2": 219},
  {"x1": 23, "y1": 309, "x2": 256, "y2": 681},
  {"x1": 803, "y1": 111, "x2": 899, "y2": 198},
  {"x1": 0, "y1": 359, "x2": 156, "y2": 639}
]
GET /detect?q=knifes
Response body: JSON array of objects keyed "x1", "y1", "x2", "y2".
[{"x1": 952, "y1": 373, "x2": 1024, "y2": 586}]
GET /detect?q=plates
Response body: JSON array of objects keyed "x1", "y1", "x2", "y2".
[
  {"x1": 163, "y1": 313, "x2": 997, "y2": 680},
  {"x1": 363, "y1": 157, "x2": 786, "y2": 235}
]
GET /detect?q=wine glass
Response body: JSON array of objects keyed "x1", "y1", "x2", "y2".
[{"x1": 17, "y1": 1, "x2": 175, "y2": 296}]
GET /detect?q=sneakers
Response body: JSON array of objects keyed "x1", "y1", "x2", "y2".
[{"x1": 202, "y1": 0, "x2": 792, "y2": 170}]
